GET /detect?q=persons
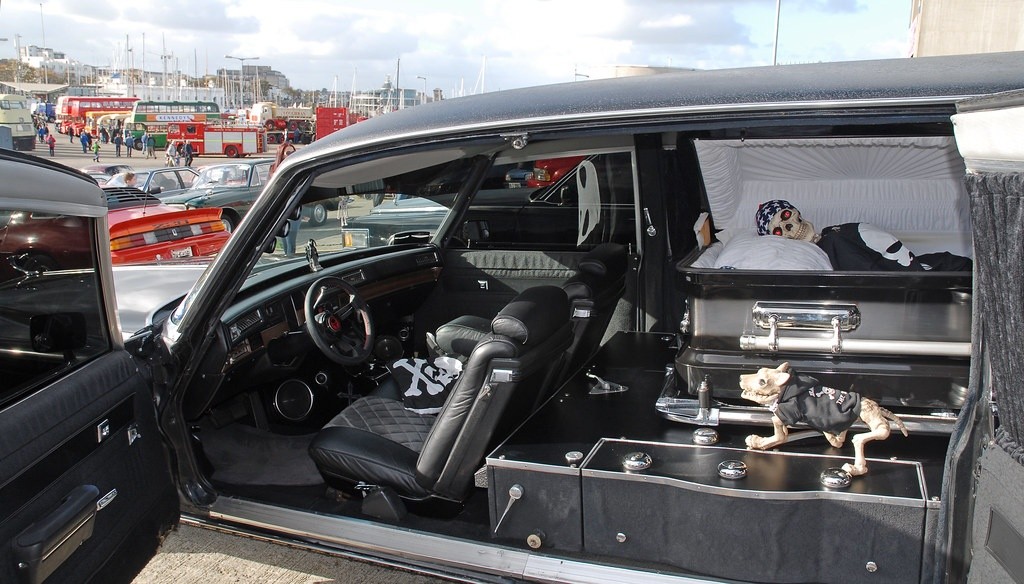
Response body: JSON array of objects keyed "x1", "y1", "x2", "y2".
[
  {"x1": 266, "y1": 142, "x2": 301, "y2": 255},
  {"x1": 293, "y1": 128, "x2": 300, "y2": 144},
  {"x1": 145, "y1": 134, "x2": 157, "y2": 159},
  {"x1": 100, "y1": 128, "x2": 108, "y2": 144},
  {"x1": 125, "y1": 133, "x2": 133, "y2": 158},
  {"x1": 46, "y1": 134, "x2": 56, "y2": 157},
  {"x1": 114, "y1": 133, "x2": 122, "y2": 157},
  {"x1": 80, "y1": 129, "x2": 93, "y2": 153},
  {"x1": 33, "y1": 117, "x2": 49, "y2": 143},
  {"x1": 141, "y1": 133, "x2": 148, "y2": 154},
  {"x1": 164, "y1": 139, "x2": 194, "y2": 168},
  {"x1": 92, "y1": 141, "x2": 101, "y2": 162},
  {"x1": 68, "y1": 125, "x2": 74, "y2": 143},
  {"x1": 124, "y1": 173, "x2": 137, "y2": 187},
  {"x1": 754, "y1": 199, "x2": 975, "y2": 271}
]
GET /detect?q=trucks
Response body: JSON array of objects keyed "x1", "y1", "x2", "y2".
[
  {"x1": 31, "y1": 103, "x2": 56, "y2": 123},
  {"x1": 0, "y1": 51, "x2": 1023, "y2": 584},
  {"x1": 0, "y1": 94, "x2": 37, "y2": 150}
]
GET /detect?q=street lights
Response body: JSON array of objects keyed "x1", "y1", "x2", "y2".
[
  {"x1": 225, "y1": 55, "x2": 260, "y2": 109},
  {"x1": 161, "y1": 54, "x2": 171, "y2": 100},
  {"x1": 417, "y1": 76, "x2": 426, "y2": 105}
]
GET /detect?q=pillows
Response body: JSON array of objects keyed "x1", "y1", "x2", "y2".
[{"x1": 714, "y1": 228, "x2": 833, "y2": 272}]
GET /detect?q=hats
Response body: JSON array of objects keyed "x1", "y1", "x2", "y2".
[{"x1": 755, "y1": 199, "x2": 795, "y2": 237}]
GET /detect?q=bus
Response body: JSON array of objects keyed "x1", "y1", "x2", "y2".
[
  {"x1": 166, "y1": 123, "x2": 267, "y2": 158},
  {"x1": 124, "y1": 100, "x2": 237, "y2": 151},
  {"x1": 55, "y1": 96, "x2": 140, "y2": 142}
]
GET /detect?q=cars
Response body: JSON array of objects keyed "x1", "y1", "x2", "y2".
[
  {"x1": 83, "y1": 160, "x2": 346, "y2": 266},
  {"x1": 343, "y1": 133, "x2": 718, "y2": 251},
  {"x1": 505, "y1": 156, "x2": 589, "y2": 188}
]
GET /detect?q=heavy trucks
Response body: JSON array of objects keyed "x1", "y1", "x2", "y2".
[{"x1": 248, "y1": 102, "x2": 368, "y2": 144}]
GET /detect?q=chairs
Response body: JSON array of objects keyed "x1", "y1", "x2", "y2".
[
  {"x1": 304, "y1": 280, "x2": 574, "y2": 499},
  {"x1": 427, "y1": 238, "x2": 632, "y2": 378},
  {"x1": 160, "y1": 178, "x2": 177, "y2": 189}
]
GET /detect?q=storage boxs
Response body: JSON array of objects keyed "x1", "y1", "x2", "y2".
[{"x1": 673, "y1": 233, "x2": 972, "y2": 355}]
